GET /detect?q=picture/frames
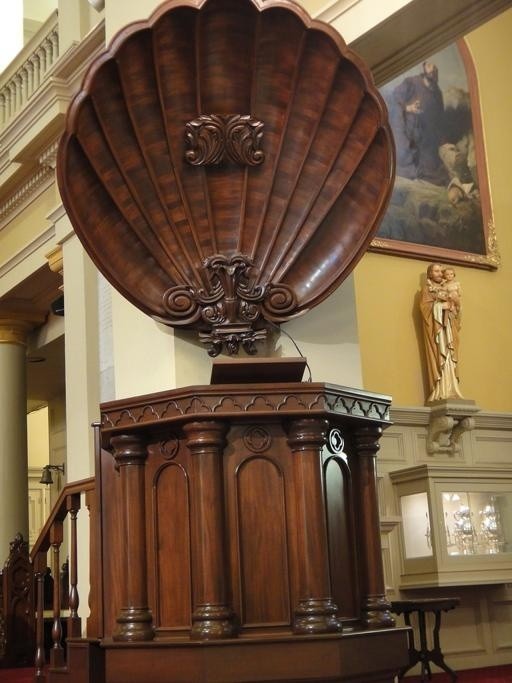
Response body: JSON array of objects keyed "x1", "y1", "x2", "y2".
[{"x1": 364, "y1": 36, "x2": 504, "y2": 273}]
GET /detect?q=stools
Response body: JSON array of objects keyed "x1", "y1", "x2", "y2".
[{"x1": 392, "y1": 597, "x2": 461, "y2": 682}]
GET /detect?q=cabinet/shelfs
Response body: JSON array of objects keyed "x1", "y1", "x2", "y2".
[{"x1": 389, "y1": 462, "x2": 512, "y2": 589}]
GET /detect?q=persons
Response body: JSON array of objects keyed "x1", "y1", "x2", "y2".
[
  {"x1": 420, "y1": 263, "x2": 464, "y2": 399},
  {"x1": 395, "y1": 60, "x2": 446, "y2": 184},
  {"x1": 430, "y1": 267, "x2": 461, "y2": 318}
]
